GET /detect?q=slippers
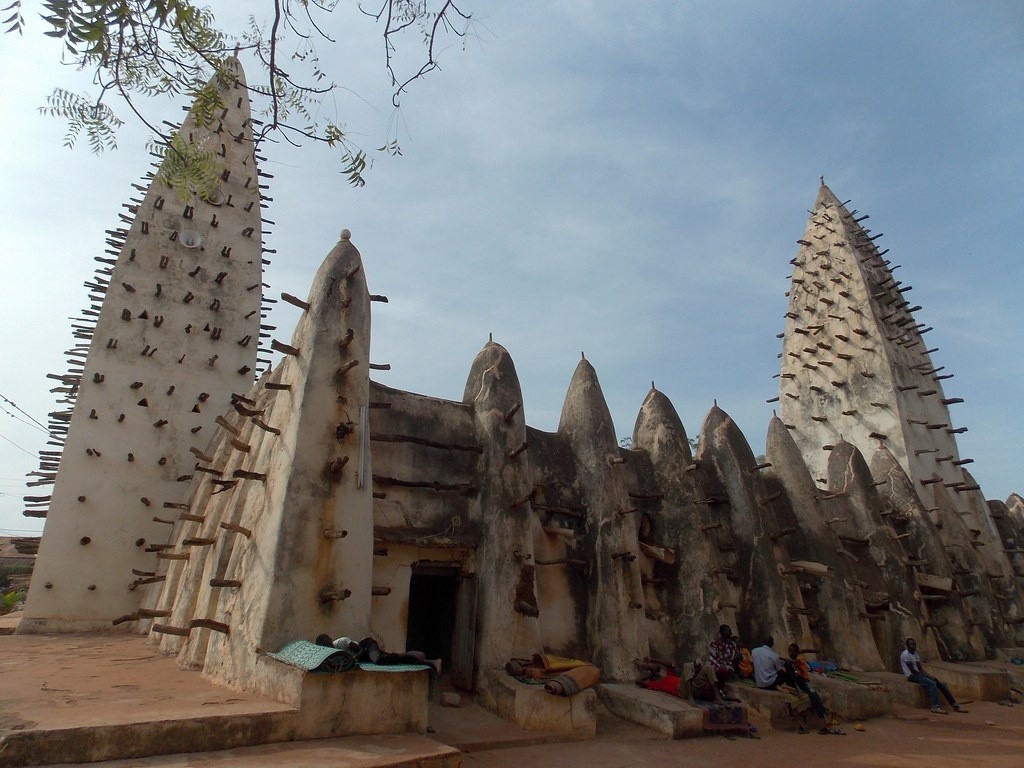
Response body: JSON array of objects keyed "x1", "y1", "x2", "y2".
[
  {"x1": 833, "y1": 727, "x2": 847, "y2": 735},
  {"x1": 818, "y1": 726, "x2": 835, "y2": 734},
  {"x1": 739, "y1": 732, "x2": 760, "y2": 739},
  {"x1": 723, "y1": 733, "x2": 736, "y2": 740}
]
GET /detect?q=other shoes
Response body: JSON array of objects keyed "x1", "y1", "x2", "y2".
[
  {"x1": 930, "y1": 706, "x2": 948, "y2": 714},
  {"x1": 953, "y1": 706, "x2": 969, "y2": 713},
  {"x1": 852, "y1": 723, "x2": 866, "y2": 731},
  {"x1": 797, "y1": 726, "x2": 809, "y2": 734}
]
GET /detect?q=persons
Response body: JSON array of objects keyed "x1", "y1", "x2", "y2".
[
  {"x1": 708, "y1": 625, "x2": 830, "y2": 725},
  {"x1": 640, "y1": 662, "x2": 729, "y2": 708},
  {"x1": 316, "y1": 634, "x2": 443, "y2": 674},
  {"x1": 901, "y1": 638, "x2": 970, "y2": 714}
]
